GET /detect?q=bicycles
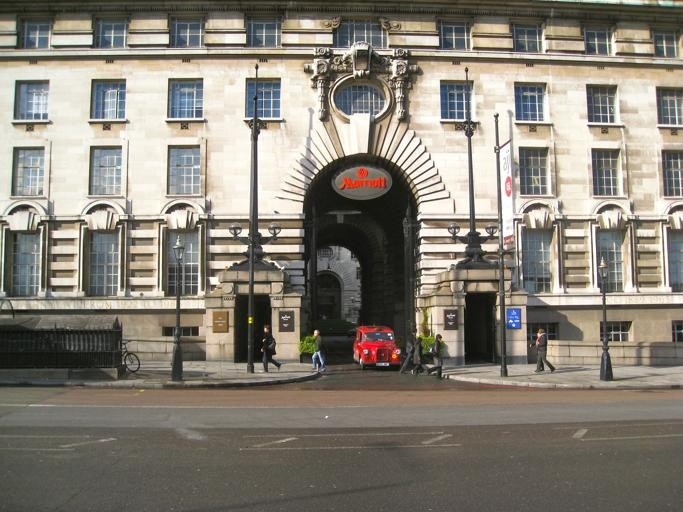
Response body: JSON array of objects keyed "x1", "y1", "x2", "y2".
[{"x1": 121, "y1": 338, "x2": 140, "y2": 372}]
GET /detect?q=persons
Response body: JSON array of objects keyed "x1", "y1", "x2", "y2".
[
  {"x1": 398, "y1": 326, "x2": 444, "y2": 381},
  {"x1": 534, "y1": 328, "x2": 555, "y2": 374},
  {"x1": 258, "y1": 324, "x2": 280, "y2": 374},
  {"x1": 310, "y1": 328, "x2": 327, "y2": 373},
  {"x1": 528, "y1": 331, "x2": 544, "y2": 372}
]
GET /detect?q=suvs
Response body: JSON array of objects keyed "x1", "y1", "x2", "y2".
[{"x1": 350, "y1": 323, "x2": 402, "y2": 372}]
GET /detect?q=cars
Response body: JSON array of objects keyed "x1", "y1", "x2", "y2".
[{"x1": 310, "y1": 318, "x2": 355, "y2": 337}]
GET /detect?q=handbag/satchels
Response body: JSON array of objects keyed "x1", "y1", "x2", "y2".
[
  {"x1": 409, "y1": 345, "x2": 415, "y2": 354},
  {"x1": 429, "y1": 341, "x2": 441, "y2": 356},
  {"x1": 267, "y1": 338, "x2": 276, "y2": 355}
]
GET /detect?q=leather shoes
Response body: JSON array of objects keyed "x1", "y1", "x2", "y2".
[
  {"x1": 534, "y1": 368, "x2": 556, "y2": 373},
  {"x1": 399, "y1": 369, "x2": 443, "y2": 380}
]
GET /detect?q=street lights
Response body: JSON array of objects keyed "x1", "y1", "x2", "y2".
[
  {"x1": 227, "y1": 62, "x2": 281, "y2": 272},
  {"x1": 445, "y1": 65, "x2": 499, "y2": 268},
  {"x1": 170, "y1": 233, "x2": 186, "y2": 381},
  {"x1": 597, "y1": 253, "x2": 616, "y2": 381}
]
